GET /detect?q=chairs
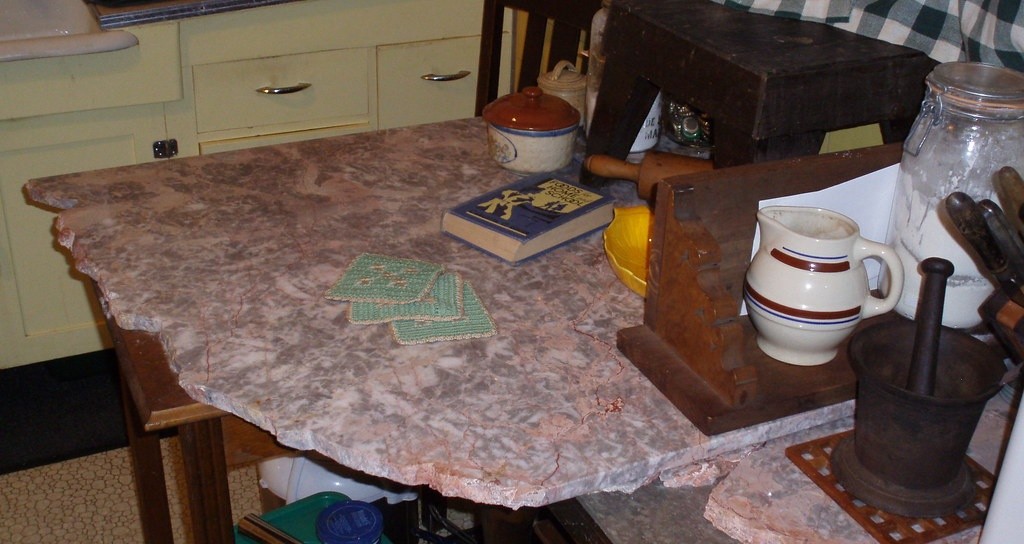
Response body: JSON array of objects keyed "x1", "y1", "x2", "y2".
[{"x1": 473, "y1": 0, "x2": 600, "y2": 118}]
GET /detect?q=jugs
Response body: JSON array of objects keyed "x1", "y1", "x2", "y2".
[{"x1": 743, "y1": 205, "x2": 905, "y2": 367}]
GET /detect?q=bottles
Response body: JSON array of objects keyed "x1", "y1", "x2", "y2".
[
  {"x1": 586, "y1": 0, "x2": 612, "y2": 141},
  {"x1": 877, "y1": 60, "x2": 1024, "y2": 329}
]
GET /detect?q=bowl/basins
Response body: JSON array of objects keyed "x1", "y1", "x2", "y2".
[
  {"x1": 480, "y1": 85, "x2": 582, "y2": 176},
  {"x1": 830, "y1": 319, "x2": 1009, "y2": 518}
]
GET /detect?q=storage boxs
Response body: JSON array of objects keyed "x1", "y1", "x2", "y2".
[{"x1": 233, "y1": 492, "x2": 392, "y2": 544}]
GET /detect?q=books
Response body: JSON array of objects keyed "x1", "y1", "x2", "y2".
[{"x1": 440, "y1": 170, "x2": 617, "y2": 266}]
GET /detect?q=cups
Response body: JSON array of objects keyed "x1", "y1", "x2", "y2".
[{"x1": 537, "y1": 60, "x2": 587, "y2": 126}]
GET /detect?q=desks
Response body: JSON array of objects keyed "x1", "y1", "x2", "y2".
[
  {"x1": 91, "y1": 281, "x2": 238, "y2": 544},
  {"x1": 583, "y1": 1, "x2": 928, "y2": 190}
]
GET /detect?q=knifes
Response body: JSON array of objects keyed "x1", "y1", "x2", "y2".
[{"x1": 945, "y1": 165, "x2": 1024, "y2": 307}]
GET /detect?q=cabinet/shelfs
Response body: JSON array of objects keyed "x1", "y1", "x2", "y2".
[{"x1": 0, "y1": 0, "x2": 513, "y2": 372}]
